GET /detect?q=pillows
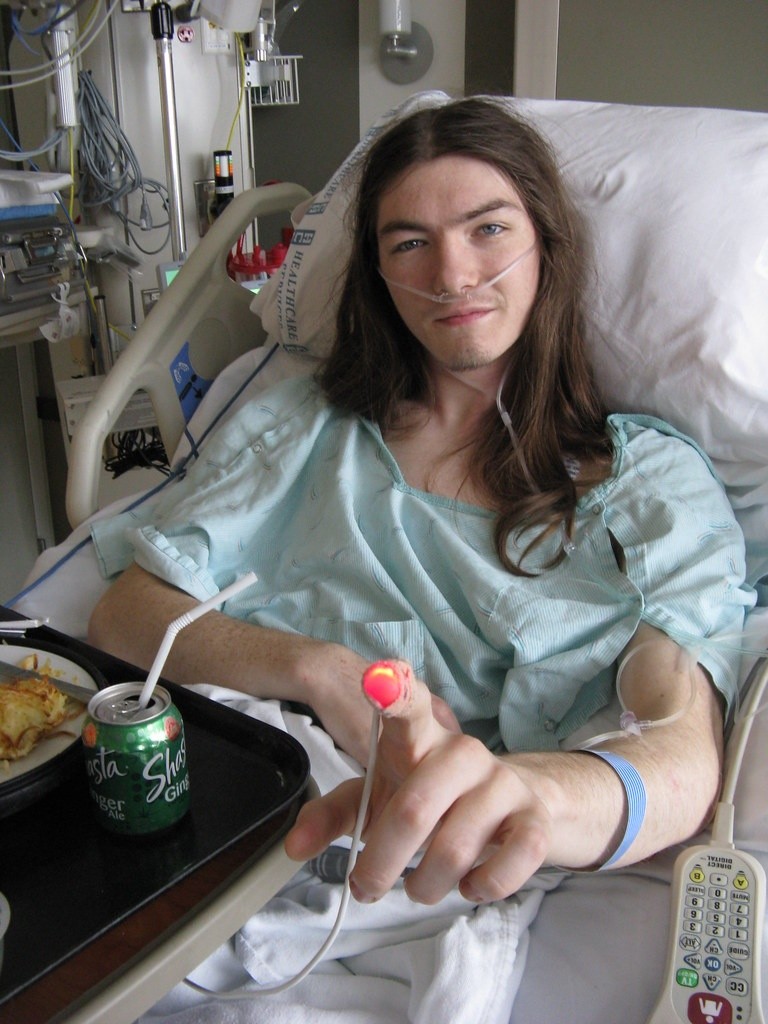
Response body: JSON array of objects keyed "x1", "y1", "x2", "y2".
[{"x1": 250, "y1": 88, "x2": 768, "y2": 464}]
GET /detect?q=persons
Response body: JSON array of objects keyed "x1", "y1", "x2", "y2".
[{"x1": 87, "y1": 97, "x2": 750, "y2": 908}]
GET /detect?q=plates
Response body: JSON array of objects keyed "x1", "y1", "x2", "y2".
[{"x1": 0, "y1": 646, "x2": 99, "y2": 784}]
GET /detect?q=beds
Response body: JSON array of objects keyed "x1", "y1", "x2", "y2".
[{"x1": 0, "y1": 184, "x2": 768, "y2": 1024}]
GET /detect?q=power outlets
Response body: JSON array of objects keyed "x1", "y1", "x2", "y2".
[
  {"x1": 176, "y1": 24, "x2": 196, "y2": 43},
  {"x1": 194, "y1": 180, "x2": 218, "y2": 237}
]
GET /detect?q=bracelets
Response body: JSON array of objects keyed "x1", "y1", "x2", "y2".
[{"x1": 548, "y1": 749, "x2": 646, "y2": 875}]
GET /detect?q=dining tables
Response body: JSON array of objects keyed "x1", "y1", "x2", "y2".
[{"x1": 0, "y1": 774, "x2": 324, "y2": 1024}]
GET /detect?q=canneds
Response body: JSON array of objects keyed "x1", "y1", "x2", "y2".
[{"x1": 79, "y1": 681, "x2": 190, "y2": 835}]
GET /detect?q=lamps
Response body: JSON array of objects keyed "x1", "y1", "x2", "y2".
[{"x1": 377, "y1": 0, "x2": 433, "y2": 85}]
